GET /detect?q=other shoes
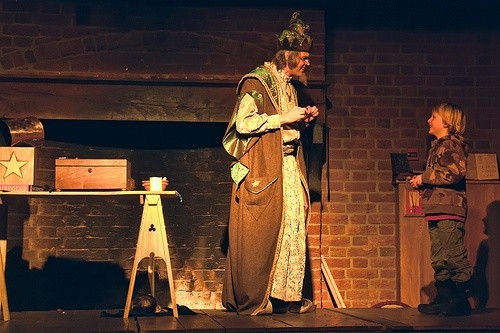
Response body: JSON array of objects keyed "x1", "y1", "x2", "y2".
[
  {"x1": 283, "y1": 297, "x2": 317, "y2": 313},
  {"x1": 257, "y1": 298, "x2": 273, "y2": 315}
]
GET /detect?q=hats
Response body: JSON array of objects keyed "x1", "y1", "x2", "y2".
[{"x1": 274, "y1": 11, "x2": 314, "y2": 53}]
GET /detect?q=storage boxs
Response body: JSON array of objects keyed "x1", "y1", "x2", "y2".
[
  {"x1": 0, "y1": 147, "x2": 37, "y2": 191},
  {"x1": 54, "y1": 158, "x2": 131, "y2": 192}
]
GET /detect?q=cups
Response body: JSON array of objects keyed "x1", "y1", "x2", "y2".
[{"x1": 150, "y1": 177, "x2": 162, "y2": 191}]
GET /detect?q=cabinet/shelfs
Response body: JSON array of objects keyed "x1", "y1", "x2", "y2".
[{"x1": 396, "y1": 181, "x2": 500, "y2": 308}]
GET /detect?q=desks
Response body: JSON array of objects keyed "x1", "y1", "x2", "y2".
[{"x1": 0, "y1": 191, "x2": 183, "y2": 321}]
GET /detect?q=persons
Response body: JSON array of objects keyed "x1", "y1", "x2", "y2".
[
  {"x1": 408, "y1": 103, "x2": 472, "y2": 317},
  {"x1": 222, "y1": 7, "x2": 320, "y2": 316}
]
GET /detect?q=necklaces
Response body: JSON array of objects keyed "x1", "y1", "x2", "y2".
[{"x1": 283, "y1": 82, "x2": 291, "y2": 102}]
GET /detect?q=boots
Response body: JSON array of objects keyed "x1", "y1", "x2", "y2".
[
  {"x1": 417, "y1": 278, "x2": 451, "y2": 315},
  {"x1": 441, "y1": 280, "x2": 472, "y2": 315}
]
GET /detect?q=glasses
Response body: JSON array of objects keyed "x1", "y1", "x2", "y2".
[{"x1": 285, "y1": 51, "x2": 313, "y2": 62}]
